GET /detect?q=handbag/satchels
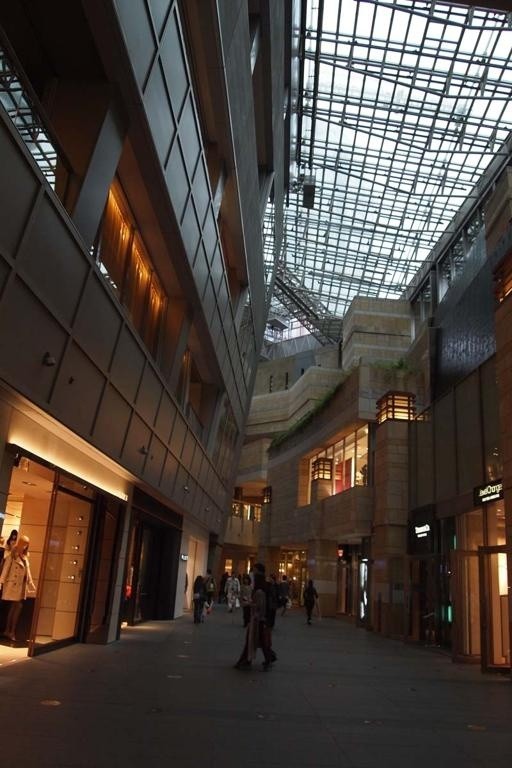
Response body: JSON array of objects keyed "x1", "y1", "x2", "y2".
[
  {"x1": 193, "y1": 592, "x2": 200, "y2": 600},
  {"x1": 235, "y1": 598, "x2": 241, "y2": 608},
  {"x1": 26, "y1": 590, "x2": 36, "y2": 598}
]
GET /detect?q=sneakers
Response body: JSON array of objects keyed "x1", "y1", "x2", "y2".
[{"x1": 234, "y1": 650, "x2": 278, "y2": 675}]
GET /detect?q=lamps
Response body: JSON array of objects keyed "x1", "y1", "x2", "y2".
[
  {"x1": 43, "y1": 355, "x2": 54, "y2": 366},
  {"x1": 141, "y1": 446, "x2": 148, "y2": 454}
]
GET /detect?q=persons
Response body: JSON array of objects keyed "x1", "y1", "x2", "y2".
[
  {"x1": 193, "y1": 567, "x2": 293, "y2": 641},
  {"x1": 234, "y1": 571, "x2": 279, "y2": 671},
  {"x1": 302, "y1": 578, "x2": 319, "y2": 625},
  {"x1": 0, "y1": 535, "x2": 37, "y2": 642},
  {"x1": 6, "y1": 530, "x2": 18, "y2": 548}
]
